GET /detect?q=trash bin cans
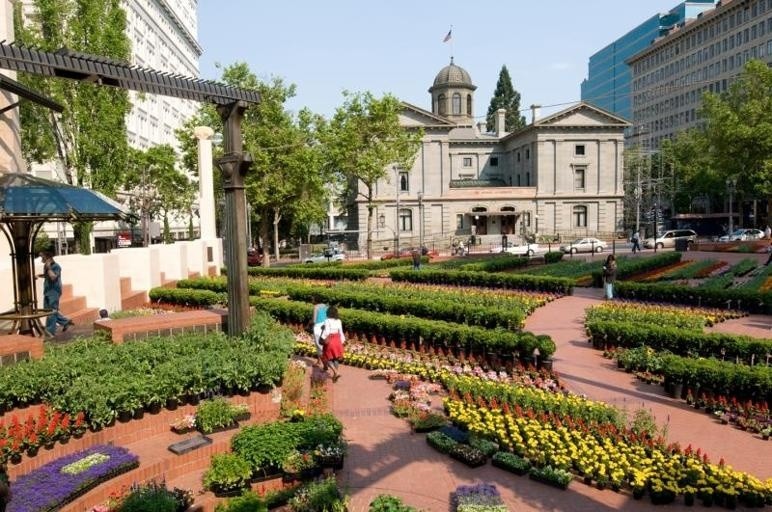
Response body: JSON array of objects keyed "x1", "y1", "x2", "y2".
[{"x1": 675, "y1": 238, "x2": 687, "y2": 251}]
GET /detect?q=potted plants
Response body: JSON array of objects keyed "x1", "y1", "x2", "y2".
[
  {"x1": 366, "y1": 375, "x2": 771, "y2": 510},
  {"x1": 0, "y1": 332, "x2": 352, "y2": 511},
  {"x1": 146, "y1": 250, "x2": 771, "y2": 377}
]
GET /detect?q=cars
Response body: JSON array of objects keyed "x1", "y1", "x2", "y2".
[
  {"x1": 490, "y1": 241, "x2": 541, "y2": 257},
  {"x1": 381, "y1": 245, "x2": 429, "y2": 259},
  {"x1": 247, "y1": 247, "x2": 261, "y2": 266},
  {"x1": 718, "y1": 228, "x2": 765, "y2": 242},
  {"x1": 559, "y1": 238, "x2": 607, "y2": 253},
  {"x1": 302, "y1": 249, "x2": 348, "y2": 264},
  {"x1": 642, "y1": 229, "x2": 698, "y2": 248}
]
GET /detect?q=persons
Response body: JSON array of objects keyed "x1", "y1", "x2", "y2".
[
  {"x1": 411, "y1": 248, "x2": 421, "y2": 271},
  {"x1": 450, "y1": 243, "x2": 457, "y2": 256},
  {"x1": 312, "y1": 294, "x2": 330, "y2": 374},
  {"x1": 318, "y1": 303, "x2": 347, "y2": 383},
  {"x1": 765, "y1": 224, "x2": 772, "y2": 240},
  {"x1": 763, "y1": 241, "x2": 772, "y2": 266},
  {"x1": 36, "y1": 250, "x2": 73, "y2": 343},
  {"x1": 604, "y1": 255, "x2": 617, "y2": 269},
  {"x1": 632, "y1": 229, "x2": 641, "y2": 251},
  {"x1": 601, "y1": 261, "x2": 617, "y2": 302},
  {"x1": 95, "y1": 308, "x2": 112, "y2": 323},
  {"x1": 462, "y1": 244, "x2": 469, "y2": 257},
  {"x1": 455, "y1": 240, "x2": 465, "y2": 257}
]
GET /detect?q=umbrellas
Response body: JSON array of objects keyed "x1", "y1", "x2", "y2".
[{"x1": 0, "y1": 172, "x2": 128, "y2": 215}]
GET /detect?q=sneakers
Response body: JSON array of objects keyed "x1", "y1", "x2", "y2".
[{"x1": 311, "y1": 364, "x2": 341, "y2": 383}]
